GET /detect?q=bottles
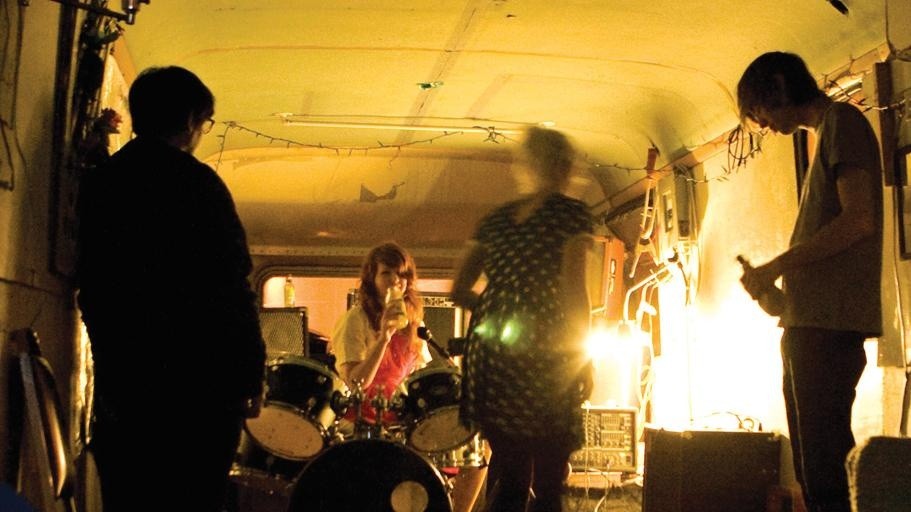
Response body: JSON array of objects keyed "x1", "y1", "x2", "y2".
[
  {"x1": 384, "y1": 286, "x2": 409, "y2": 330},
  {"x1": 283, "y1": 274, "x2": 296, "y2": 309},
  {"x1": 736, "y1": 254, "x2": 787, "y2": 316}
]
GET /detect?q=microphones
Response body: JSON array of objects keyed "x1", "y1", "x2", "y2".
[{"x1": 413, "y1": 324, "x2": 452, "y2": 365}]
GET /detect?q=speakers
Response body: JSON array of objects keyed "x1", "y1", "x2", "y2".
[{"x1": 640, "y1": 425, "x2": 783, "y2": 512}]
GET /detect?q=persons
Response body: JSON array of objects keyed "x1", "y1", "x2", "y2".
[
  {"x1": 70, "y1": 66, "x2": 267, "y2": 512},
  {"x1": 736, "y1": 50, "x2": 884, "y2": 512},
  {"x1": 330, "y1": 239, "x2": 434, "y2": 435},
  {"x1": 448, "y1": 125, "x2": 593, "y2": 512}
]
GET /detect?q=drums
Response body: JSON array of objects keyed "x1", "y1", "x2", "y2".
[
  {"x1": 242, "y1": 356, "x2": 352, "y2": 460},
  {"x1": 283, "y1": 440, "x2": 455, "y2": 511},
  {"x1": 228, "y1": 430, "x2": 307, "y2": 512},
  {"x1": 433, "y1": 431, "x2": 486, "y2": 470},
  {"x1": 390, "y1": 368, "x2": 479, "y2": 454}
]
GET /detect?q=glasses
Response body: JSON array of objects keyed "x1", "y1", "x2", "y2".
[{"x1": 199, "y1": 117, "x2": 215, "y2": 134}]
